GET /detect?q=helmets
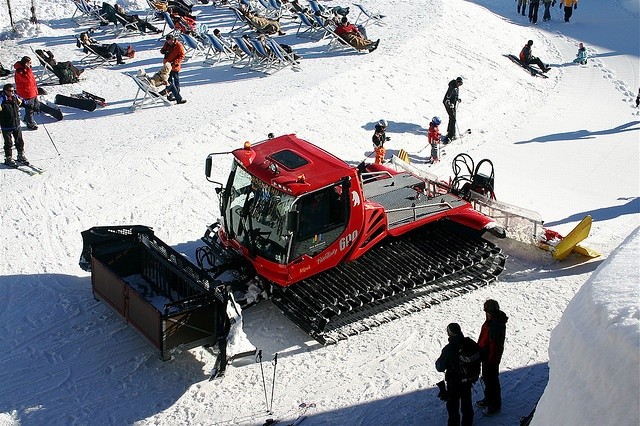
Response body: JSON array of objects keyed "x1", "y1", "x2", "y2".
[
  {"x1": 378, "y1": 119, "x2": 388, "y2": 131},
  {"x1": 432, "y1": 117, "x2": 441, "y2": 125}
]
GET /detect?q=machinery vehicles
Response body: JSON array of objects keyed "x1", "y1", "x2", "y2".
[{"x1": 79, "y1": 132, "x2": 599, "y2": 382}]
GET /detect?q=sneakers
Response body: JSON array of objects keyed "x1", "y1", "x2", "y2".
[
  {"x1": 369, "y1": 39, "x2": 380, "y2": 52},
  {"x1": 27, "y1": 122, "x2": 38, "y2": 130},
  {"x1": 176, "y1": 98, "x2": 187, "y2": 104},
  {"x1": 167, "y1": 95, "x2": 176, "y2": 100},
  {"x1": 127, "y1": 45, "x2": 135, "y2": 58},
  {"x1": 117, "y1": 61, "x2": 125, "y2": 65},
  {"x1": 482, "y1": 406, "x2": 502, "y2": 416},
  {"x1": 543, "y1": 67, "x2": 551, "y2": 73},
  {"x1": 475, "y1": 398, "x2": 488, "y2": 407},
  {"x1": 77, "y1": 69, "x2": 84, "y2": 76}
]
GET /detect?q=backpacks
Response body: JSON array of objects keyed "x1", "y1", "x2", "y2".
[{"x1": 448, "y1": 337, "x2": 481, "y2": 378}]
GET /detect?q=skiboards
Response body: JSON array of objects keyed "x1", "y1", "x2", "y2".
[
  {"x1": 443, "y1": 129, "x2": 471, "y2": 149},
  {"x1": 263, "y1": 403, "x2": 316, "y2": 426},
  {"x1": 505, "y1": 54, "x2": 548, "y2": 78},
  {"x1": 5, "y1": 159, "x2": 44, "y2": 176},
  {"x1": 422, "y1": 150, "x2": 446, "y2": 168},
  {"x1": 71, "y1": 90, "x2": 106, "y2": 107}
]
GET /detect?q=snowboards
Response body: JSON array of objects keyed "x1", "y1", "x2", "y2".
[{"x1": 55, "y1": 95, "x2": 96, "y2": 112}]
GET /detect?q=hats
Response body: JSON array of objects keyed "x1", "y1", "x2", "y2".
[
  {"x1": 447, "y1": 323, "x2": 461, "y2": 338},
  {"x1": 242, "y1": 35, "x2": 250, "y2": 38}
]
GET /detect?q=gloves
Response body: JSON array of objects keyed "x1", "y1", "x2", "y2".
[
  {"x1": 162, "y1": 49, "x2": 169, "y2": 54},
  {"x1": 552, "y1": 1, "x2": 556, "y2": 7},
  {"x1": 559, "y1": 3, "x2": 562, "y2": 9},
  {"x1": 574, "y1": 4, "x2": 577, "y2": 9}
]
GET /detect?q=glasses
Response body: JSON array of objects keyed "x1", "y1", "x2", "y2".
[{"x1": 5, "y1": 89, "x2": 14, "y2": 92}]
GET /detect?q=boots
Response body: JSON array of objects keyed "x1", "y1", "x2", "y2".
[
  {"x1": 17, "y1": 155, "x2": 29, "y2": 165},
  {"x1": 5, "y1": 156, "x2": 19, "y2": 168}
]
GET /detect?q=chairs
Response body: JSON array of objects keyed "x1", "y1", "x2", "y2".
[
  {"x1": 30, "y1": 45, "x2": 61, "y2": 83},
  {"x1": 172, "y1": 0, "x2": 389, "y2": 80},
  {"x1": 74, "y1": 35, "x2": 115, "y2": 69},
  {"x1": 72, "y1": 0, "x2": 172, "y2": 41},
  {"x1": 126, "y1": 71, "x2": 173, "y2": 109}
]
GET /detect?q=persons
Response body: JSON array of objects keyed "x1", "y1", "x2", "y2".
[
  {"x1": 240, "y1": 5, "x2": 287, "y2": 35},
  {"x1": 316, "y1": 10, "x2": 334, "y2": 27},
  {"x1": 13, "y1": 56, "x2": 39, "y2": 130},
  {"x1": 302, "y1": 8, "x2": 319, "y2": 27},
  {"x1": 243, "y1": 35, "x2": 257, "y2": 51},
  {"x1": 514, "y1": 0, "x2": 527, "y2": 16},
  {"x1": 90, "y1": 37, "x2": 136, "y2": 58},
  {"x1": 527, "y1": 0, "x2": 539, "y2": 25},
  {"x1": 372, "y1": 119, "x2": 391, "y2": 164},
  {"x1": 573, "y1": 43, "x2": 587, "y2": 65},
  {"x1": 560, "y1": 0, "x2": 577, "y2": 22},
  {"x1": 0, "y1": 84, "x2": 39, "y2": 166},
  {"x1": 114, "y1": 4, "x2": 163, "y2": 34},
  {"x1": 151, "y1": 0, "x2": 195, "y2": 19},
  {"x1": 436, "y1": 323, "x2": 483, "y2": 426},
  {"x1": 541, "y1": 0, "x2": 557, "y2": 22},
  {"x1": 520, "y1": 40, "x2": 551, "y2": 73},
  {"x1": 136, "y1": 68, "x2": 151, "y2": 80},
  {"x1": 257, "y1": 36, "x2": 300, "y2": 65},
  {"x1": 476, "y1": 299, "x2": 508, "y2": 416},
  {"x1": 151, "y1": 62, "x2": 187, "y2": 104},
  {"x1": 335, "y1": 16, "x2": 380, "y2": 52},
  {"x1": 214, "y1": 28, "x2": 238, "y2": 51},
  {"x1": 81, "y1": 32, "x2": 127, "y2": 64},
  {"x1": 333, "y1": 16, "x2": 367, "y2": 39},
  {"x1": 36, "y1": 49, "x2": 84, "y2": 78},
  {"x1": 428, "y1": 117, "x2": 442, "y2": 161},
  {"x1": 444, "y1": 77, "x2": 464, "y2": 140},
  {"x1": 161, "y1": 35, "x2": 185, "y2": 101}
]
